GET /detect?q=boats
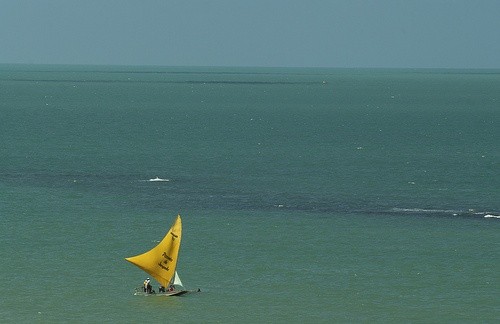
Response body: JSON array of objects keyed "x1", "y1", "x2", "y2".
[
  {"x1": 149, "y1": 176, "x2": 171, "y2": 182},
  {"x1": 483, "y1": 215, "x2": 500, "y2": 219}
]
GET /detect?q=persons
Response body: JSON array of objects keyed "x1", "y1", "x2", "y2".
[{"x1": 143, "y1": 277, "x2": 176, "y2": 295}]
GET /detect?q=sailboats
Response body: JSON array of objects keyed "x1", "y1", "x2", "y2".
[{"x1": 123, "y1": 214, "x2": 201, "y2": 297}]
